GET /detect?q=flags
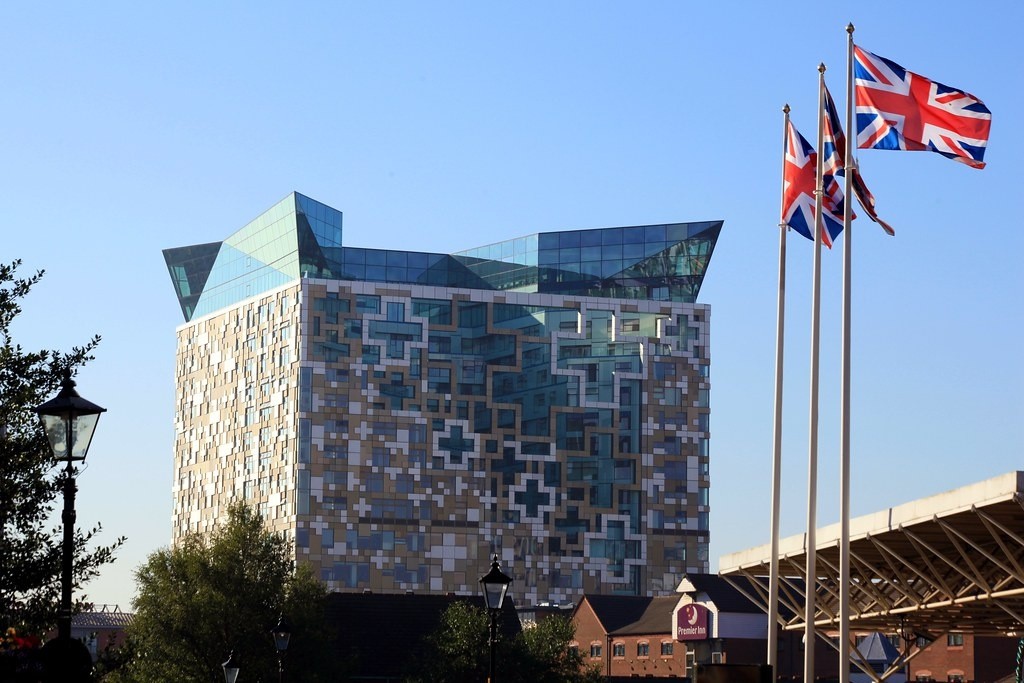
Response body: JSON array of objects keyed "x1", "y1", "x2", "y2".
[
  {"x1": 853, "y1": 45, "x2": 993, "y2": 170},
  {"x1": 782, "y1": 117, "x2": 857, "y2": 250},
  {"x1": 823, "y1": 87, "x2": 894, "y2": 236}
]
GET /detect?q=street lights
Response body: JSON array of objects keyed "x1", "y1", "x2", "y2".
[
  {"x1": 268, "y1": 610, "x2": 293, "y2": 683},
  {"x1": 32, "y1": 367, "x2": 108, "y2": 683},
  {"x1": 477, "y1": 553, "x2": 512, "y2": 683}
]
